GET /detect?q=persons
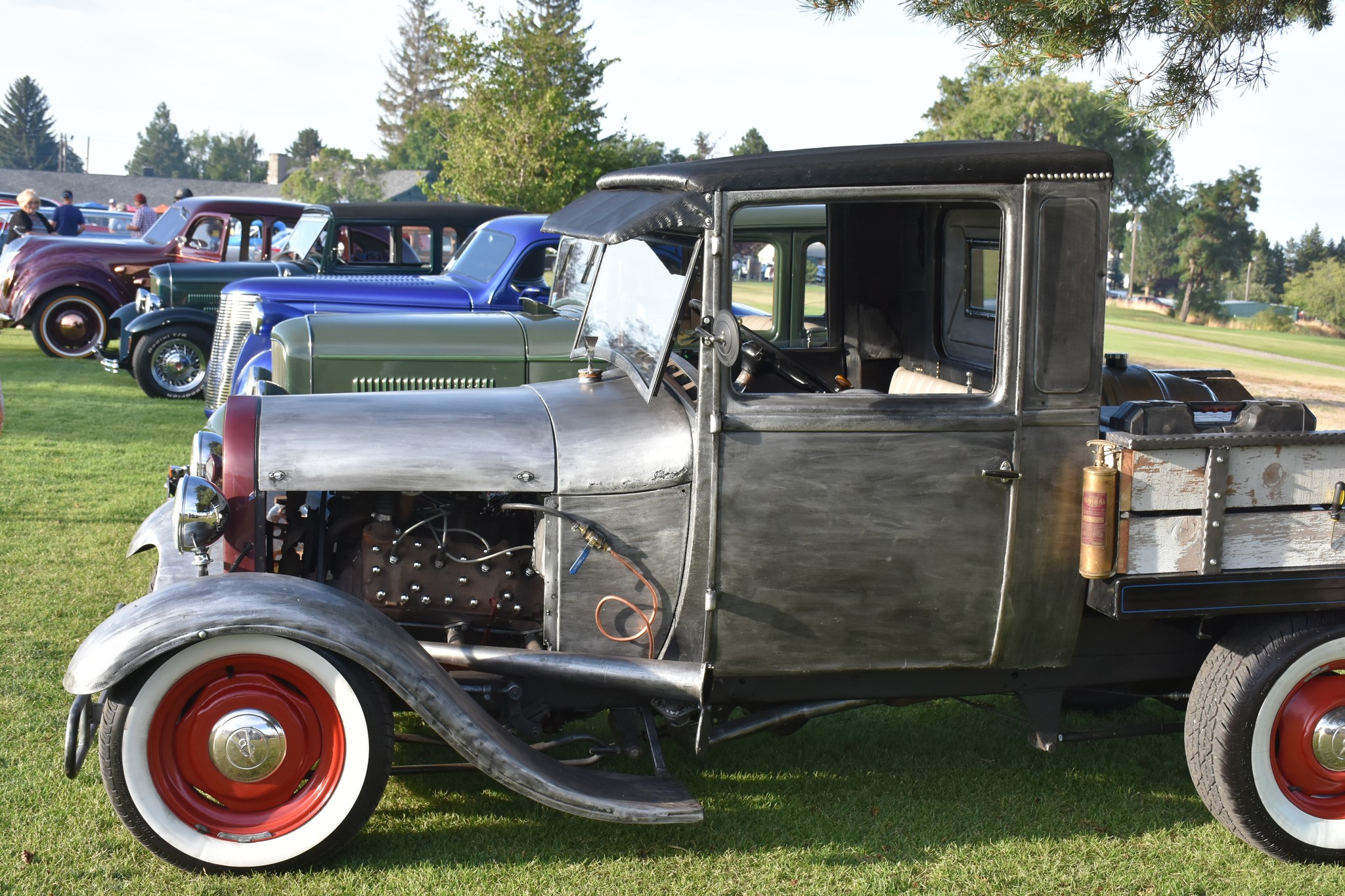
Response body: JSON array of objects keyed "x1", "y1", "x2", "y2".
[
  {"x1": 109, "y1": 204, "x2": 132, "y2": 234},
  {"x1": 732, "y1": 256, "x2": 739, "y2": 280},
  {"x1": 48, "y1": 190, "x2": 85, "y2": 237},
  {"x1": 6, "y1": 189, "x2": 59, "y2": 245},
  {"x1": 174, "y1": 188, "x2": 192, "y2": 203},
  {"x1": 108, "y1": 199, "x2": 118, "y2": 212},
  {"x1": 127, "y1": 193, "x2": 158, "y2": 238}
]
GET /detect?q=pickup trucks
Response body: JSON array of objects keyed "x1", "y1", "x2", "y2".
[
  {"x1": 0, "y1": 194, "x2": 816, "y2": 411},
  {"x1": 56, "y1": 139, "x2": 1345, "y2": 878}
]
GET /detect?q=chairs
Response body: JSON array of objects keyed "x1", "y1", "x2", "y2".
[{"x1": 882, "y1": 366, "x2": 988, "y2": 396}]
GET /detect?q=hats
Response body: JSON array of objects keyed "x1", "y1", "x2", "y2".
[
  {"x1": 63, "y1": 191, "x2": 73, "y2": 198},
  {"x1": 174, "y1": 188, "x2": 193, "y2": 200},
  {"x1": 109, "y1": 198, "x2": 115, "y2": 203},
  {"x1": 134, "y1": 193, "x2": 146, "y2": 201}
]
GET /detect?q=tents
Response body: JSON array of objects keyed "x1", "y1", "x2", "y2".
[
  {"x1": 125, "y1": 204, "x2": 137, "y2": 213},
  {"x1": 73, "y1": 201, "x2": 108, "y2": 226},
  {"x1": 153, "y1": 204, "x2": 169, "y2": 213}
]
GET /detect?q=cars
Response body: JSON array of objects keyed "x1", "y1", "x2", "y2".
[
  {"x1": 732, "y1": 251, "x2": 774, "y2": 283},
  {"x1": 811, "y1": 265, "x2": 826, "y2": 284}
]
